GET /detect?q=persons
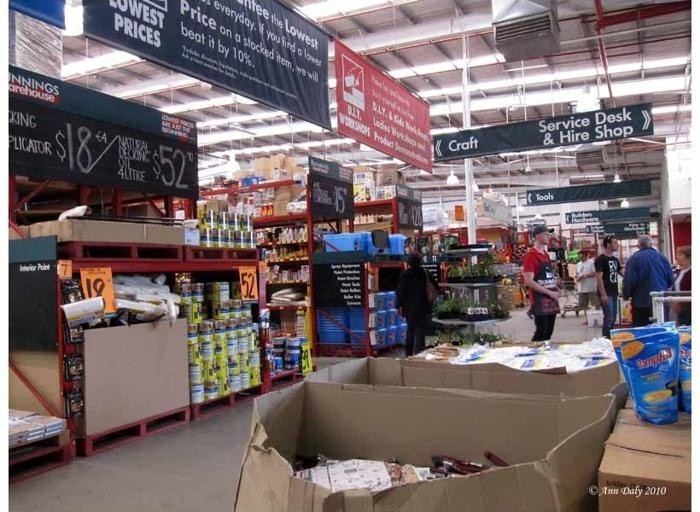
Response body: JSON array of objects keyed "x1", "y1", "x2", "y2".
[
  {"x1": 395, "y1": 251, "x2": 442, "y2": 358},
  {"x1": 522, "y1": 225, "x2": 600, "y2": 341},
  {"x1": 595, "y1": 233, "x2": 624, "y2": 338},
  {"x1": 667, "y1": 246, "x2": 691, "y2": 325},
  {"x1": 619, "y1": 234, "x2": 673, "y2": 325}
]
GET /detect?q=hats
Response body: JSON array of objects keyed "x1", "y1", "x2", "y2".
[{"x1": 533, "y1": 227, "x2": 554, "y2": 237}]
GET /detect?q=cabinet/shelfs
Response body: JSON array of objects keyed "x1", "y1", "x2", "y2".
[{"x1": 667, "y1": 206, "x2": 691, "y2": 270}]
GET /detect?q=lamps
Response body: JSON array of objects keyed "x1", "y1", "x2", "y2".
[
  {"x1": 446, "y1": 161, "x2": 459, "y2": 185},
  {"x1": 521, "y1": 60, "x2": 531, "y2": 173},
  {"x1": 576, "y1": 85, "x2": 600, "y2": 113},
  {"x1": 613, "y1": 141, "x2": 622, "y2": 183},
  {"x1": 61, "y1": 0, "x2": 84, "y2": 36}
]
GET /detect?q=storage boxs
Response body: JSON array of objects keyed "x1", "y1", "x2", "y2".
[{"x1": 255, "y1": 154, "x2": 306, "y2": 180}]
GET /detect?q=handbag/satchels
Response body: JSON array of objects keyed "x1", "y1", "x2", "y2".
[{"x1": 427, "y1": 283, "x2": 436, "y2": 304}]
